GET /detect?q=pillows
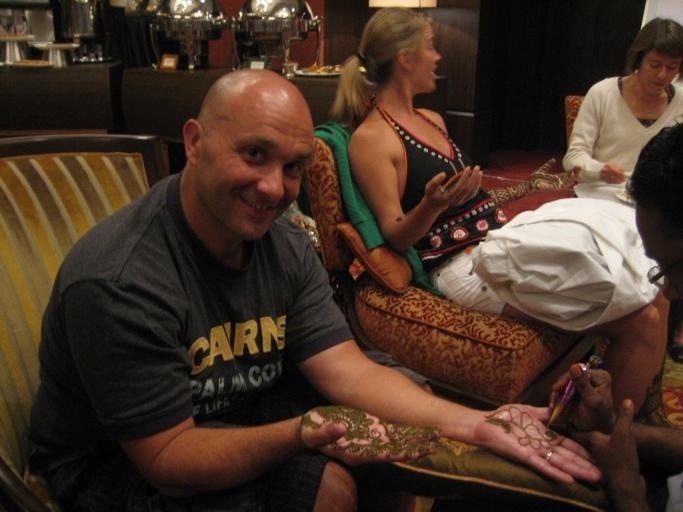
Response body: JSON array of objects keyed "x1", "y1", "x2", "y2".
[
  {"x1": 335, "y1": 221, "x2": 415, "y2": 294},
  {"x1": 387, "y1": 426, "x2": 612, "y2": 512}
]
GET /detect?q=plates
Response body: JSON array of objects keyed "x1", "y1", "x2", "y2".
[
  {"x1": 615, "y1": 191, "x2": 637, "y2": 204},
  {"x1": 6, "y1": 59, "x2": 55, "y2": 70},
  {"x1": 294, "y1": 63, "x2": 367, "y2": 78}
]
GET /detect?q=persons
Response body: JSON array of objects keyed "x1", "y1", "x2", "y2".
[
  {"x1": 27, "y1": 70, "x2": 604, "y2": 509},
  {"x1": 545, "y1": 123, "x2": 683, "y2": 511},
  {"x1": 326, "y1": 3, "x2": 675, "y2": 426},
  {"x1": 564, "y1": 17, "x2": 683, "y2": 208}
]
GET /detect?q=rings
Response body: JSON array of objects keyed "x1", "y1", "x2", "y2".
[
  {"x1": 546, "y1": 451, "x2": 555, "y2": 462},
  {"x1": 609, "y1": 175, "x2": 614, "y2": 180}
]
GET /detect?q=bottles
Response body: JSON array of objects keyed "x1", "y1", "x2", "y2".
[{"x1": 289, "y1": 203, "x2": 306, "y2": 230}]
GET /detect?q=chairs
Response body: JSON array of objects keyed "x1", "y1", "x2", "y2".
[
  {"x1": 302, "y1": 107, "x2": 603, "y2": 409},
  {"x1": 0, "y1": 133, "x2": 170, "y2": 512},
  {"x1": 565, "y1": 94, "x2": 586, "y2": 150}
]
